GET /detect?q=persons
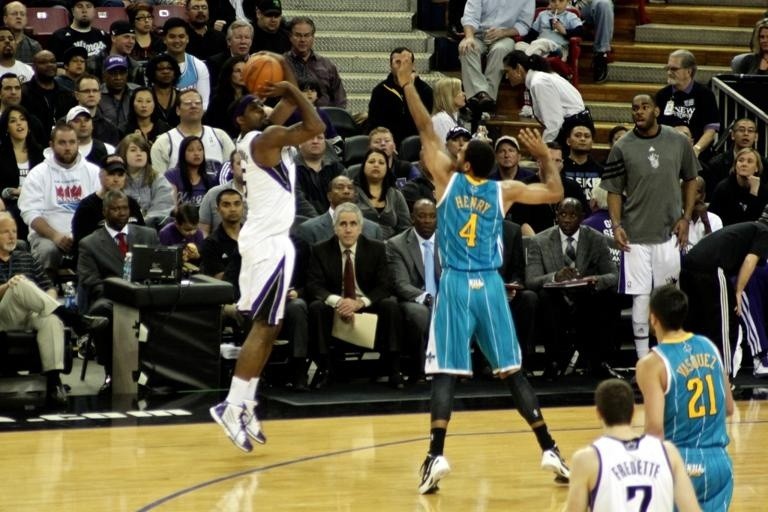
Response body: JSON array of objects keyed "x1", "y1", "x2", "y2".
[
  {"x1": 391, "y1": 50, "x2": 571, "y2": 493},
  {"x1": 563, "y1": 379, "x2": 702, "y2": 512},
  {"x1": 2, "y1": 0, "x2": 768, "y2": 401},
  {"x1": 207, "y1": 51, "x2": 328, "y2": 453},
  {"x1": 634, "y1": 285, "x2": 734, "y2": 512}
]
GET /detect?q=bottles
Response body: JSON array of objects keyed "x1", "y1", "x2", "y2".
[
  {"x1": 120, "y1": 250, "x2": 133, "y2": 283},
  {"x1": 63, "y1": 280, "x2": 77, "y2": 308}
]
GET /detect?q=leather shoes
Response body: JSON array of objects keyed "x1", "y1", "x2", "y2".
[
  {"x1": 591, "y1": 54, "x2": 609, "y2": 82},
  {"x1": 47, "y1": 385, "x2": 70, "y2": 408},
  {"x1": 597, "y1": 361, "x2": 625, "y2": 381}
]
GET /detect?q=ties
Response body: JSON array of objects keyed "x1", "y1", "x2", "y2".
[
  {"x1": 117, "y1": 234, "x2": 128, "y2": 254},
  {"x1": 423, "y1": 242, "x2": 436, "y2": 293},
  {"x1": 344, "y1": 250, "x2": 355, "y2": 299},
  {"x1": 566, "y1": 236, "x2": 576, "y2": 260}
]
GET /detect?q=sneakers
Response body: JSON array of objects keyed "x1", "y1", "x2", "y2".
[
  {"x1": 77, "y1": 338, "x2": 95, "y2": 359},
  {"x1": 540, "y1": 449, "x2": 569, "y2": 479},
  {"x1": 419, "y1": 455, "x2": 450, "y2": 493},
  {"x1": 753, "y1": 357, "x2": 768, "y2": 377},
  {"x1": 293, "y1": 371, "x2": 311, "y2": 393},
  {"x1": 100, "y1": 374, "x2": 111, "y2": 393},
  {"x1": 730, "y1": 382, "x2": 752, "y2": 400},
  {"x1": 470, "y1": 93, "x2": 496, "y2": 111},
  {"x1": 209, "y1": 400, "x2": 254, "y2": 452},
  {"x1": 64, "y1": 305, "x2": 79, "y2": 326},
  {"x1": 242, "y1": 401, "x2": 266, "y2": 445}
]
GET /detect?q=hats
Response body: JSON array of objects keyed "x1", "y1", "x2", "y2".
[
  {"x1": 103, "y1": 154, "x2": 127, "y2": 173},
  {"x1": 446, "y1": 126, "x2": 473, "y2": 140},
  {"x1": 494, "y1": 135, "x2": 520, "y2": 152},
  {"x1": 104, "y1": 55, "x2": 128, "y2": 71},
  {"x1": 63, "y1": 46, "x2": 87, "y2": 61},
  {"x1": 258, "y1": 0, "x2": 281, "y2": 16},
  {"x1": 65, "y1": 105, "x2": 92, "y2": 122}
]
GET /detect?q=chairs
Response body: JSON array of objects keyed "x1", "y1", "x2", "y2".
[{"x1": 0, "y1": 0, "x2": 768, "y2": 404}]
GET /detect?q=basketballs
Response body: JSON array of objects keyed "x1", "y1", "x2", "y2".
[{"x1": 242, "y1": 54, "x2": 283, "y2": 98}]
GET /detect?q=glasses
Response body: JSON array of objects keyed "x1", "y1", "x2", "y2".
[{"x1": 135, "y1": 15, "x2": 153, "y2": 21}]
